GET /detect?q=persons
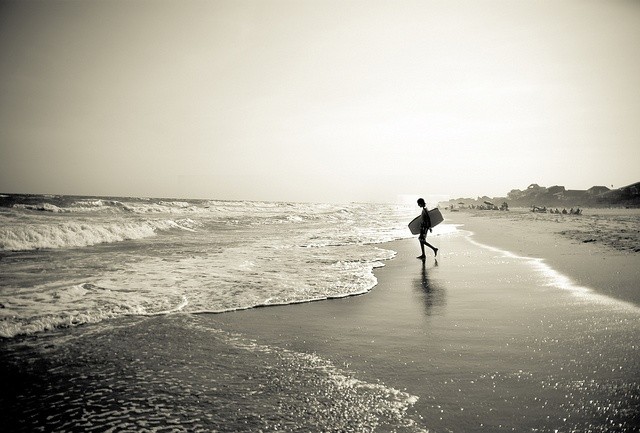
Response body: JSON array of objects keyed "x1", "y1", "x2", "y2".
[
  {"x1": 416, "y1": 198, "x2": 438, "y2": 259},
  {"x1": 492, "y1": 202, "x2": 509, "y2": 211},
  {"x1": 528, "y1": 206, "x2": 581, "y2": 215},
  {"x1": 468, "y1": 204, "x2": 485, "y2": 209}
]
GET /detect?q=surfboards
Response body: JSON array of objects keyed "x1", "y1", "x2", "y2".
[{"x1": 408, "y1": 208, "x2": 444, "y2": 234}]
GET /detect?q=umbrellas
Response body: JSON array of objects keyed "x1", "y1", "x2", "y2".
[
  {"x1": 458, "y1": 202, "x2": 464, "y2": 204},
  {"x1": 484, "y1": 201, "x2": 493, "y2": 206}
]
